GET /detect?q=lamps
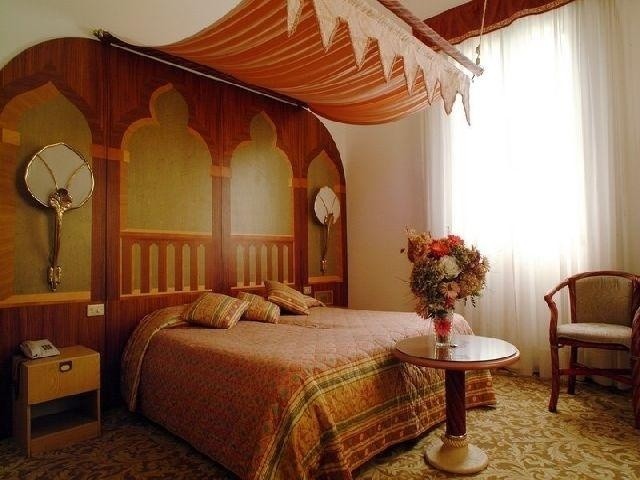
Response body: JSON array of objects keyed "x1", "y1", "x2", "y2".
[
  {"x1": 22, "y1": 142, "x2": 95, "y2": 291},
  {"x1": 312, "y1": 187, "x2": 340, "y2": 274}
]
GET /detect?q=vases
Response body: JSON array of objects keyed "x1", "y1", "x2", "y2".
[{"x1": 434, "y1": 308, "x2": 454, "y2": 348}]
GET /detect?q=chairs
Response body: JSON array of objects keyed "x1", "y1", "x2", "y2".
[{"x1": 545, "y1": 271, "x2": 640, "y2": 429}]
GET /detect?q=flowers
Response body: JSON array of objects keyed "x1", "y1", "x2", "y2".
[{"x1": 404, "y1": 228, "x2": 490, "y2": 342}]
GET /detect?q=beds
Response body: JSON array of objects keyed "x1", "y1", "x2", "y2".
[{"x1": 118, "y1": 293, "x2": 497, "y2": 479}]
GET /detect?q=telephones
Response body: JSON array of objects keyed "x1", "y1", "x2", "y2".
[{"x1": 20, "y1": 339, "x2": 60, "y2": 359}]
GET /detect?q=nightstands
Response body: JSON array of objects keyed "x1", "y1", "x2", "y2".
[{"x1": 11, "y1": 346, "x2": 102, "y2": 458}]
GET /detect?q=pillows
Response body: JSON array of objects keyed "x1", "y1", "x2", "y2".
[{"x1": 157, "y1": 277, "x2": 319, "y2": 329}]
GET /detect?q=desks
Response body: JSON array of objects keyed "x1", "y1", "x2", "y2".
[{"x1": 392, "y1": 335, "x2": 520, "y2": 474}]
